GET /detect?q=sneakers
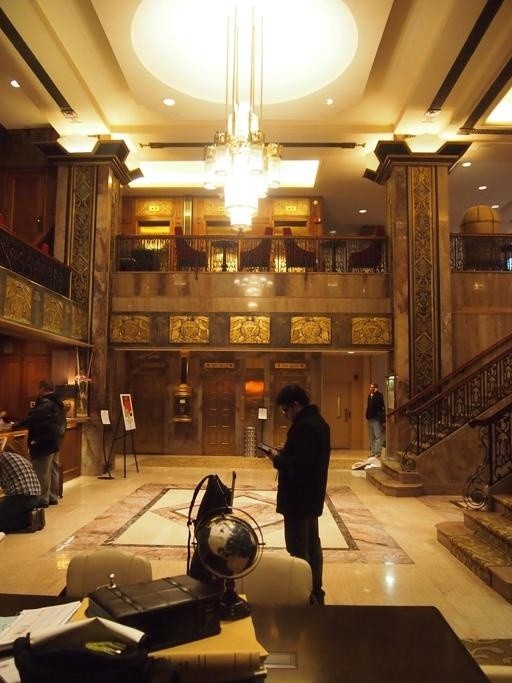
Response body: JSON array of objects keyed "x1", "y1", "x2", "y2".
[{"x1": 40, "y1": 499, "x2": 59, "y2": 507}]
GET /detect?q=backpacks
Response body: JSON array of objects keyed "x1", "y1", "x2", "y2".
[{"x1": 188, "y1": 473, "x2": 232, "y2": 539}]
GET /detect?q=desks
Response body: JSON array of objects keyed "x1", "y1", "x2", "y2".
[
  {"x1": 318, "y1": 233, "x2": 353, "y2": 270},
  {"x1": 209, "y1": 239, "x2": 238, "y2": 271},
  {"x1": 131, "y1": 247, "x2": 162, "y2": 270},
  {"x1": 2, "y1": 585, "x2": 496, "y2": 681}
]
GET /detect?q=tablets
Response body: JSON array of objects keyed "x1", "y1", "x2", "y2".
[{"x1": 256, "y1": 440, "x2": 282, "y2": 457}]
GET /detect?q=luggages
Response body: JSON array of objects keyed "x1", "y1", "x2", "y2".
[{"x1": 189, "y1": 546, "x2": 225, "y2": 591}]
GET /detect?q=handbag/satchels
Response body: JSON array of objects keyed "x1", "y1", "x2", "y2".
[{"x1": 377, "y1": 410, "x2": 386, "y2": 423}]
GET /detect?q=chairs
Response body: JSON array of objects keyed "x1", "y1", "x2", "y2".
[
  {"x1": 238, "y1": 224, "x2": 275, "y2": 273},
  {"x1": 345, "y1": 224, "x2": 386, "y2": 272},
  {"x1": 235, "y1": 546, "x2": 319, "y2": 609},
  {"x1": 278, "y1": 226, "x2": 317, "y2": 271},
  {"x1": 57, "y1": 552, "x2": 159, "y2": 602},
  {"x1": 170, "y1": 221, "x2": 212, "y2": 271}
]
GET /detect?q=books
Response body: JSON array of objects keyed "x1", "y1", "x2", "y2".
[{"x1": 0, "y1": 592, "x2": 269, "y2": 682}]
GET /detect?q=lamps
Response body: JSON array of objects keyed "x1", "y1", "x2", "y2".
[{"x1": 196, "y1": 0, "x2": 286, "y2": 234}]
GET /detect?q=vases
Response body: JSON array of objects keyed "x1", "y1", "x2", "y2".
[{"x1": 76, "y1": 391, "x2": 89, "y2": 418}]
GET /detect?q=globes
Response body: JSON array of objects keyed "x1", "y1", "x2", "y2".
[{"x1": 192, "y1": 506, "x2": 265, "y2": 620}]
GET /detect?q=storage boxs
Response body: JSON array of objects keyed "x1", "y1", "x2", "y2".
[{"x1": 83, "y1": 574, "x2": 224, "y2": 655}]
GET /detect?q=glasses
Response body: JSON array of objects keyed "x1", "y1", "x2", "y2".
[{"x1": 279, "y1": 401, "x2": 294, "y2": 415}]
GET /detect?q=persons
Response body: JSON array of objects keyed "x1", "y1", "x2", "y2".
[
  {"x1": 264, "y1": 381, "x2": 334, "y2": 606},
  {"x1": 363, "y1": 381, "x2": 386, "y2": 459},
  {"x1": 11, "y1": 379, "x2": 66, "y2": 508},
  {"x1": 1, "y1": 449, "x2": 47, "y2": 534}
]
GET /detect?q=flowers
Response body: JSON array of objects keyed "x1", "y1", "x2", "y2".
[{"x1": 75, "y1": 374, "x2": 93, "y2": 414}]
